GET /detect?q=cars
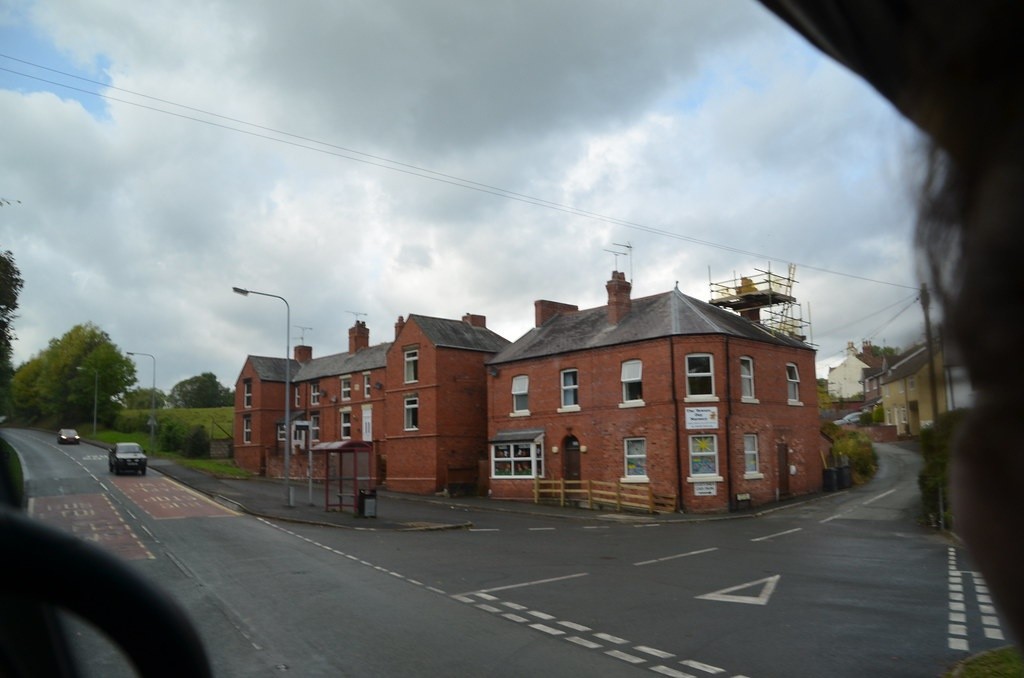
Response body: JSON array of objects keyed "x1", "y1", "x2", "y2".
[
  {"x1": 109, "y1": 442, "x2": 148, "y2": 475},
  {"x1": 833, "y1": 412, "x2": 863, "y2": 425},
  {"x1": 57, "y1": 429, "x2": 80, "y2": 445}
]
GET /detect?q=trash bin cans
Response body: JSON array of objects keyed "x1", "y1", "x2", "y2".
[
  {"x1": 358, "y1": 488, "x2": 378, "y2": 518},
  {"x1": 822, "y1": 468, "x2": 838, "y2": 492},
  {"x1": 837, "y1": 463, "x2": 853, "y2": 490}
]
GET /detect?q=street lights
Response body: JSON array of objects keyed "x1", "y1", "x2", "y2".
[
  {"x1": 77, "y1": 367, "x2": 98, "y2": 435},
  {"x1": 126, "y1": 352, "x2": 156, "y2": 449},
  {"x1": 232, "y1": 285, "x2": 291, "y2": 505}
]
